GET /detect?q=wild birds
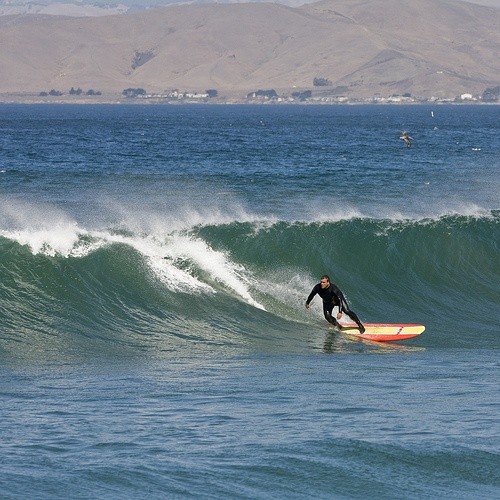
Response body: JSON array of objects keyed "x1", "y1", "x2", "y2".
[{"x1": 400, "y1": 130, "x2": 412, "y2": 147}]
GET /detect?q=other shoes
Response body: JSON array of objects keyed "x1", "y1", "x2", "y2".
[
  {"x1": 335, "y1": 324, "x2": 342, "y2": 330},
  {"x1": 357, "y1": 320, "x2": 365, "y2": 334}
]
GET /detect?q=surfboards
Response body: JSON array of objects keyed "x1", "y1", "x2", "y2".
[{"x1": 336, "y1": 322, "x2": 426, "y2": 342}]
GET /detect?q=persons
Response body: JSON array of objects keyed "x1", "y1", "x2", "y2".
[{"x1": 305, "y1": 275, "x2": 365, "y2": 334}]
